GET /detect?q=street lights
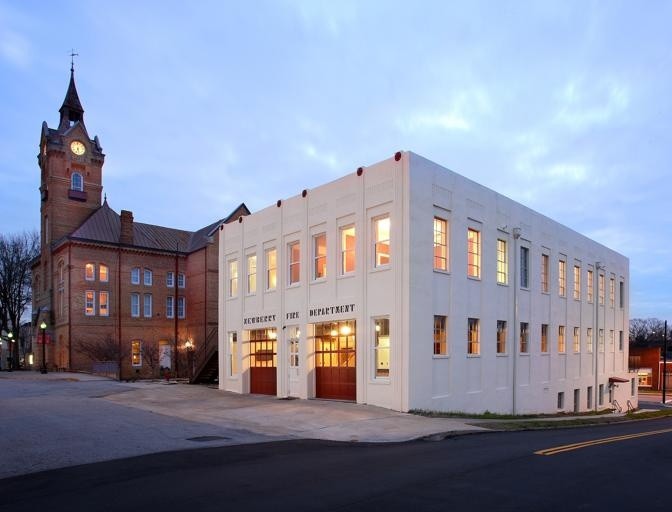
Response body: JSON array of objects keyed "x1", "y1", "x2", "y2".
[
  {"x1": 40, "y1": 321, "x2": 48, "y2": 374},
  {"x1": 8, "y1": 331, "x2": 14, "y2": 372}
]
